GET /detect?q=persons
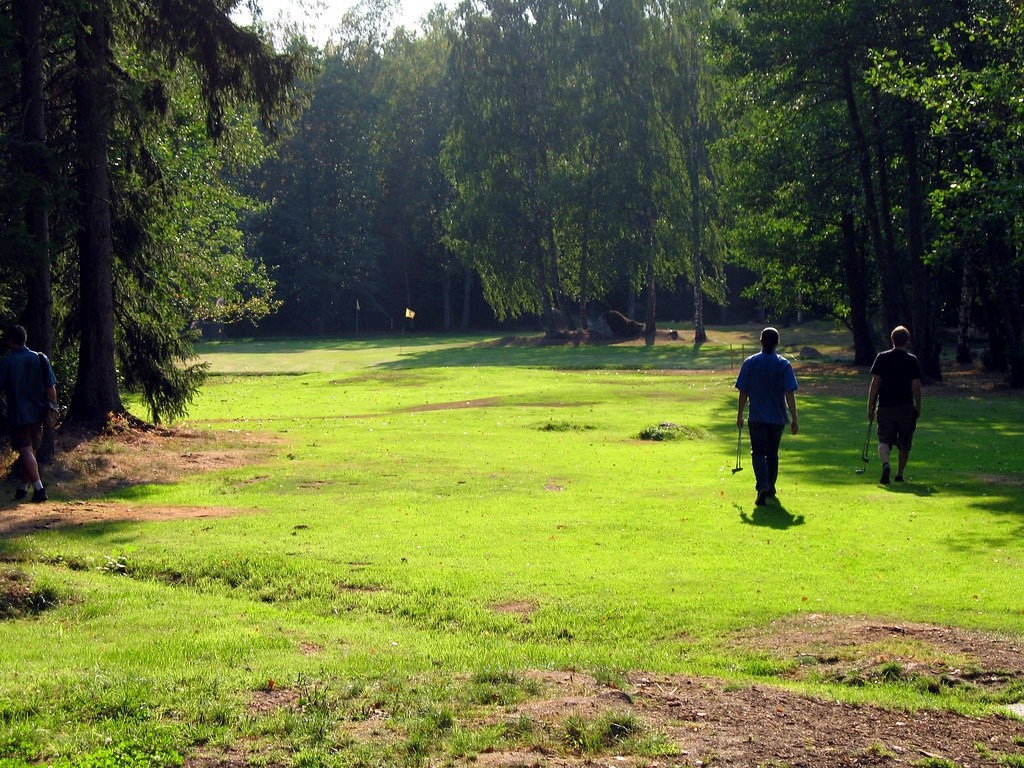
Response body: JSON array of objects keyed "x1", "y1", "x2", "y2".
[
  {"x1": 1, "y1": 325, "x2": 60, "y2": 503},
  {"x1": 735, "y1": 327, "x2": 799, "y2": 507},
  {"x1": 867, "y1": 325, "x2": 921, "y2": 484}
]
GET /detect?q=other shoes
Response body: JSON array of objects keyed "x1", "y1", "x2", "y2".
[
  {"x1": 880, "y1": 466, "x2": 891, "y2": 484},
  {"x1": 30, "y1": 487, "x2": 48, "y2": 502},
  {"x1": 894, "y1": 473, "x2": 904, "y2": 482},
  {"x1": 754, "y1": 485, "x2": 777, "y2": 505},
  {"x1": 14, "y1": 483, "x2": 31, "y2": 499}
]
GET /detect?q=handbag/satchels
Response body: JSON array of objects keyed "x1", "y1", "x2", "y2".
[{"x1": 44, "y1": 395, "x2": 61, "y2": 426}]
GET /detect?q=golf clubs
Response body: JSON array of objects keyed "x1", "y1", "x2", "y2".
[
  {"x1": 855, "y1": 420, "x2": 873, "y2": 474},
  {"x1": 731, "y1": 428, "x2": 743, "y2": 474}
]
